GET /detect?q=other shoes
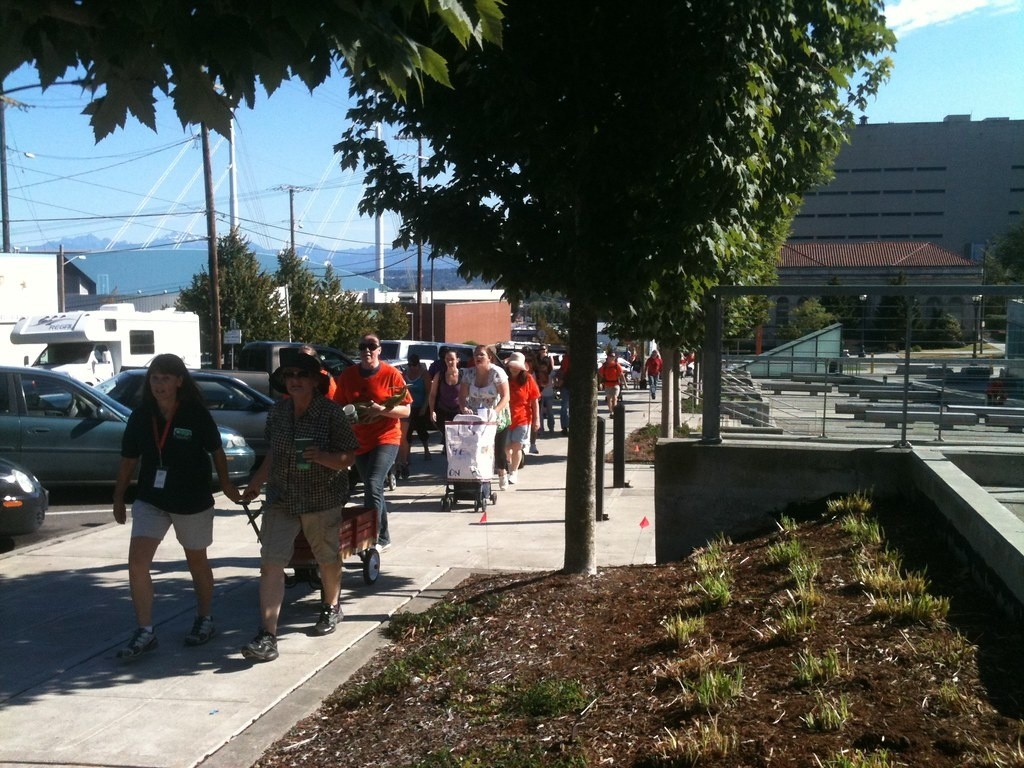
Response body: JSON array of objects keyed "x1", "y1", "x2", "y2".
[
  {"x1": 529, "y1": 446, "x2": 538, "y2": 453},
  {"x1": 652, "y1": 393, "x2": 655, "y2": 399},
  {"x1": 537, "y1": 429, "x2": 545, "y2": 435},
  {"x1": 425, "y1": 453, "x2": 432, "y2": 460},
  {"x1": 395, "y1": 462, "x2": 409, "y2": 480},
  {"x1": 379, "y1": 540, "x2": 387, "y2": 545},
  {"x1": 550, "y1": 429, "x2": 554, "y2": 433}
]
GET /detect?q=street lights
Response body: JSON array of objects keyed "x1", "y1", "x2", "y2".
[
  {"x1": 58, "y1": 243, "x2": 87, "y2": 313},
  {"x1": 858, "y1": 294, "x2": 867, "y2": 358},
  {"x1": 0, "y1": 141, "x2": 36, "y2": 253},
  {"x1": 971, "y1": 294, "x2": 983, "y2": 358}
]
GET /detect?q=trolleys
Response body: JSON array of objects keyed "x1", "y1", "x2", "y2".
[{"x1": 441, "y1": 405, "x2": 497, "y2": 513}]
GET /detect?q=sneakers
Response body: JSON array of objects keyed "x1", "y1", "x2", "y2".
[
  {"x1": 508, "y1": 472, "x2": 518, "y2": 484},
  {"x1": 313, "y1": 602, "x2": 344, "y2": 636},
  {"x1": 498, "y1": 471, "x2": 508, "y2": 490},
  {"x1": 116, "y1": 626, "x2": 159, "y2": 658},
  {"x1": 241, "y1": 626, "x2": 279, "y2": 660},
  {"x1": 185, "y1": 615, "x2": 217, "y2": 645}
]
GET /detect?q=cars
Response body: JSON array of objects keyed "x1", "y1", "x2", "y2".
[
  {"x1": 328, "y1": 338, "x2": 695, "y2": 419},
  {"x1": 0, "y1": 457, "x2": 48, "y2": 537},
  {"x1": 0, "y1": 364, "x2": 256, "y2": 504},
  {"x1": 66, "y1": 367, "x2": 276, "y2": 472}
]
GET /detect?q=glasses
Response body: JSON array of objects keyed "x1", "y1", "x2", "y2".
[
  {"x1": 407, "y1": 362, "x2": 419, "y2": 367},
  {"x1": 282, "y1": 370, "x2": 314, "y2": 379},
  {"x1": 358, "y1": 343, "x2": 379, "y2": 351}
]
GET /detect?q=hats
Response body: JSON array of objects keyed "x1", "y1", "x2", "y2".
[
  {"x1": 269, "y1": 352, "x2": 331, "y2": 396},
  {"x1": 505, "y1": 352, "x2": 528, "y2": 371}
]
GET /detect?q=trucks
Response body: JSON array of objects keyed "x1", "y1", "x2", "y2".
[{"x1": 9, "y1": 303, "x2": 201, "y2": 409}]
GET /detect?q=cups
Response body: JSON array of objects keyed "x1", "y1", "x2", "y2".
[
  {"x1": 342, "y1": 404, "x2": 359, "y2": 424},
  {"x1": 293, "y1": 438, "x2": 313, "y2": 469},
  {"x1": 477, "y1": 407, "x2": 488, "y2": 422}
]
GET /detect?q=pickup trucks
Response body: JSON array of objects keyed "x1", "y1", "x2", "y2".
[{"x1": 119, "y1": 341, "x2": 358, "y2": 402}]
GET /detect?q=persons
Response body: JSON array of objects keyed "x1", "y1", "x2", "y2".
[
  {"x1": 644, "y1": 350, "x2": 661, "y2": 399},
  {"x1": 113, "y1": 353, "x2": 242, "y2": 659},
  {"x1": 283, "y1": 344, "x2": 337, "y2": 400},
  {"x1": 680, "y1": 351, "x2": 695, "y2": 376},
  {"x1": 401, "y1": 346, "x2": 570, "y2": 492},
  {"x1": 598, "y1": 352, "x2": 628, "y2": 418},
  {"x1": 631, "y1": 356, "x2": 642, "y2": 389},
  {"x1": 332, "y1": 333, "x2": 413, "y2": 552},
  {"x1": 240, "y1": 349, "x2": 359, "y2": 661}
]
